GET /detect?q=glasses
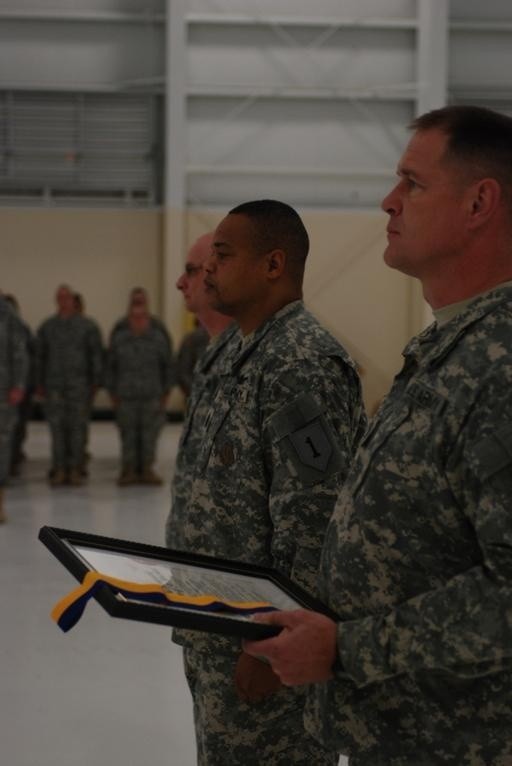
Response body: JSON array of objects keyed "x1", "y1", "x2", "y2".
[{"x1": 185, "y1": 263, "x2": 203, "y2": 276}]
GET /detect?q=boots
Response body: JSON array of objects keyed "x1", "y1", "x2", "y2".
[
  {"x1": 50, "y1": 464, "x2": 85, "y2": 486},
  {"x1": 120, "y1": 458, "x2": 159, "y2": 484}
]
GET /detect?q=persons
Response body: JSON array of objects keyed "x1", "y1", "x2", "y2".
[
  {"x1": 2, "y1": 283, "x2": 210, "y2": 487},
  {"x1": 238, "y1": 106, "x2": 512, "y2": 766},
  {"x1": 165, "y1": 199, "x2": 371, "y2": 764},
  {"x1": 160, "y1": 228, "x2": 242, "y2": 550}
]
